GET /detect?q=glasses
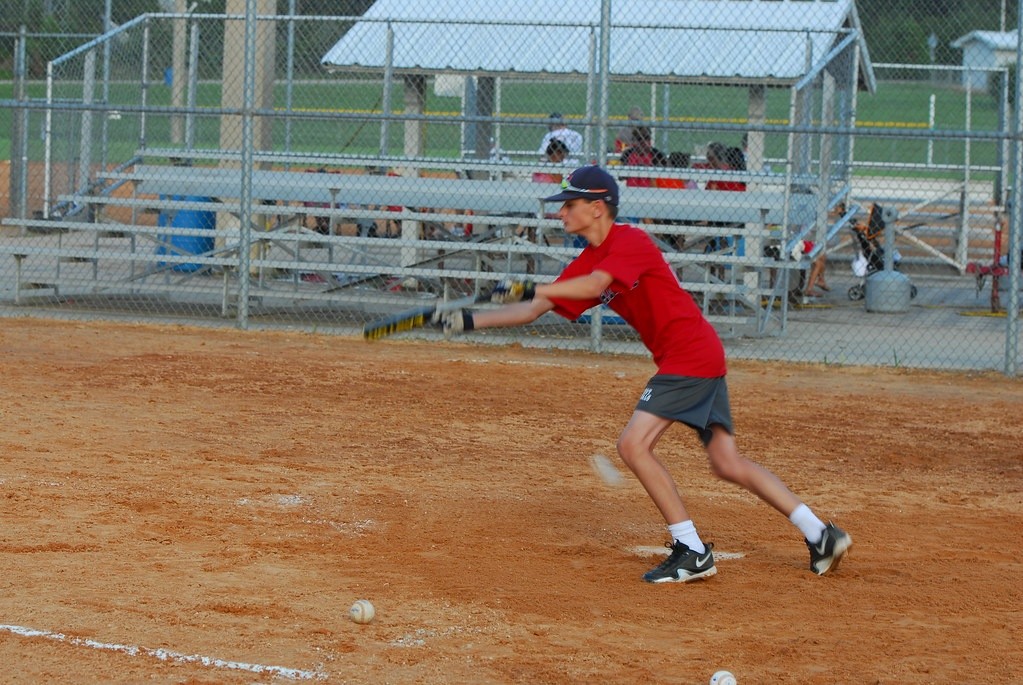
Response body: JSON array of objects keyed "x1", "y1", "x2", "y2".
[{"x1": 561, "y1": 173, "x2": 608, "y2": 193}]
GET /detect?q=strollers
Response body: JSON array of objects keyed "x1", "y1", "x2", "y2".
[{"x1": 839, "y1": 202, "x2": 917, "y2": 301}]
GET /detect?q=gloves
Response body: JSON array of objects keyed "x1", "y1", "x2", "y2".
[
  {"x1": 491, "y1": 277, "x2": 536, "y2": 303},
  {"x1": 432, "y1": 307, "x2": 474, "y2": 337}
]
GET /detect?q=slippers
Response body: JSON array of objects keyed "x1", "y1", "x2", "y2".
[
  {"x1": 805, "y1": 291, "x2": 822, "y2": 298},
  {"x1": 816, "y1": 282, "x2": 830, "y2": 291}
]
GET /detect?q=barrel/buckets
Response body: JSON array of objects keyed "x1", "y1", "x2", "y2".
[
  {"x1": 564, "y1": 217, "x2": 640, "y2": 326},
  {"x1": 153, "y1": 194, "x2": 217, "y2": 276}
]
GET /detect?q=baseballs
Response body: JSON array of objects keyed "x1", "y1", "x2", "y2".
[{"x1": 350, "y1": 599, "x2": 375, "y2": 623}]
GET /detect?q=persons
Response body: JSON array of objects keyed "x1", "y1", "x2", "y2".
[
  {"x1": 741, "y1": 133, "x2": 771, "y2": 172},
  {"x1": 616, "y1": 106, "x2": 650, "y2": 153},
  {"x1": 532, "y1": 137, "x2": 569, "y2": 182},
  {"x1": 617, "y1": 126, "x2": 667, "y2": 234},
  {"x1": 667, "y1": 151, "x2": 698, "y2": 189},
  {"x1": 539, "y1": 112, "x2": 583, "y2": 163},
  {"x1": 432, "y1": 165, "x2": 853, "y2": 583},
  {"x1": 693, "y1": 142, "x2": 832, "y2": 299}
]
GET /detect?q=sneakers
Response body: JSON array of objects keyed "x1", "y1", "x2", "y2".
[
  {"x1": 641, "y1": 539, "x2": 717, "y2": 584},
  {"x1": 805, "y1": 519, "x2": 853, "y2": 575}
]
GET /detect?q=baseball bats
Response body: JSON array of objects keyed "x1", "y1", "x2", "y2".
[{"x1": 361, "y1": 279, "x2": 534, "y2": 345}]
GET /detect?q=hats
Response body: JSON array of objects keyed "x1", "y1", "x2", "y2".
[{"x1": 543, "y1": 164, "x2": 618, "y2": 206}]
